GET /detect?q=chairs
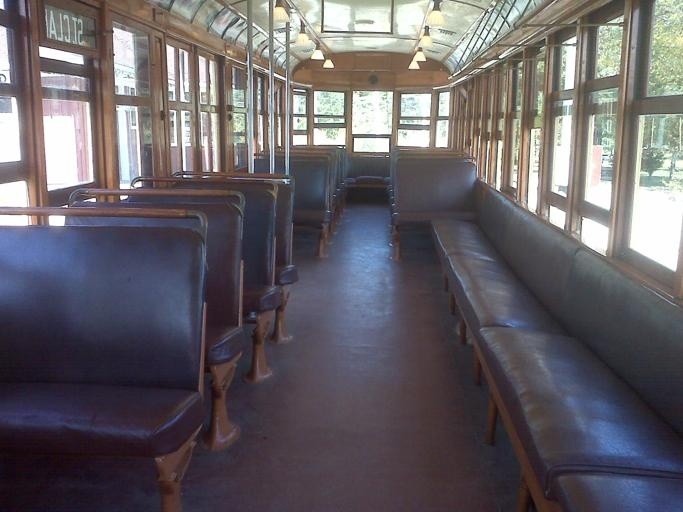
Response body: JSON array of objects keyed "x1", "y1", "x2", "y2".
[{"x1": 348, "y1": 154, "x2": 386, "y2": 191}]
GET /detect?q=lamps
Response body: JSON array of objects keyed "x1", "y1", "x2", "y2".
[
  {"x1": 296, "y1": 22, "x2": 309, "y2": 49},
  {"x1": 271, "y1": 2, "x2": 290, "y2": 22},
  {"x1": 311, "y1": 44, "x2": 324, "y2": 60},
  {"x1": 419, "y1": 26, "x2": 435, "y2": 47},
  {"x1": 426, "y1": 2, "x2": 444, "y2": 26},
  {"x1": 408, "y1": 57, "x2": 419, "y2": 70},
  {"x1": 413, "y1": 47, "x2": 427, "y2": 62},
  {"x1": 322, "y1": 55, "x2": 334, "y2": 70}
]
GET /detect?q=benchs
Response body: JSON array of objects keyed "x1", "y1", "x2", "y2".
[
  {"x1": 430, "y1": 177, "x2": 682, "y2": 510},
  {"x1": 245, "y1": 145, "x2": 346, "y2": 257},
  {"x1": 387, "y1": 144, "x2": 478, "y2": 261},
  {"x1": 66, "y1": 171, "x2": 304, "y2": 454},
  {"x1": 1, "y1": 207, "x2": 212, "y2": 512}
]
